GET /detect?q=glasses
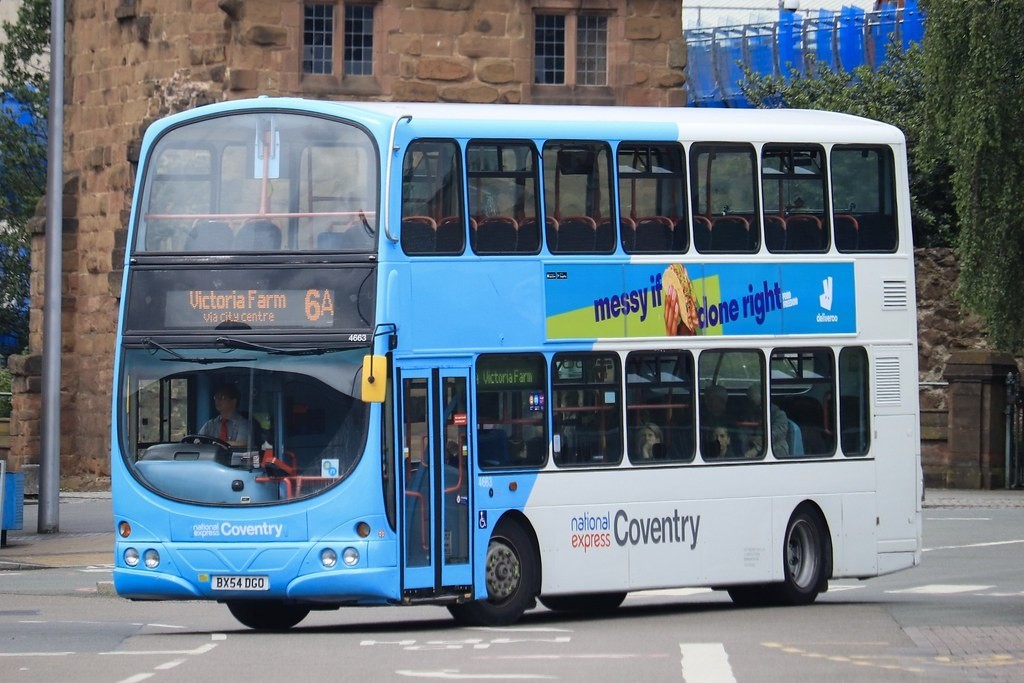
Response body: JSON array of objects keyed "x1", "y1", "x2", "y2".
[{"x1": 213, "y1": 394, "x2": 231, "y2": 400}]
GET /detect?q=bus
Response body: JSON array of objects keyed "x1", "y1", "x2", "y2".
[{"x1": 111, "y1": 96, "x2": 921, "y2": 631}]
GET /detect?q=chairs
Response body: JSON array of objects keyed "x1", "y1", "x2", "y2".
[
  {"x1": 475, "y1": 393, "x2": 832, "y2": 468},
  {"x1": 179, "y1": 213, "x2": 860, "y2": 251}
]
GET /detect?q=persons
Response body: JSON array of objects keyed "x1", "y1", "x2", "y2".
[
  {"x1": 699, "y1": 385, "x2": 740, "y2": 459},
  {"x1": 198, "y1": 385, "x2": 255, "y2": 447},
  {"x1": 560, "y1": 390, "x2": 581, "y2": 461},
  {"x1": 743, "y1": 383, "x2": 790, "y2": 459},
  {"x1": 632, "y1": 422, "x2": 664, "y2": 461},
  {"x1": 445, "y1": 440, "x2": 467, "y2": 470}
]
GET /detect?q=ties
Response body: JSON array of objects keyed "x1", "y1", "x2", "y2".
[{"x1": 220, "y1": 418, "x2": 228, "y2": 441}]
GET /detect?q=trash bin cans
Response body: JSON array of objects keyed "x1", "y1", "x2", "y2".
[{"x1": 0, "y1": 469, "x2": 25, "y2": 548}]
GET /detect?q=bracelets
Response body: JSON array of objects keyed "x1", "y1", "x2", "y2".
[{"x1": 753, "y1": 445, "x2": 760, "y2": 453}]
